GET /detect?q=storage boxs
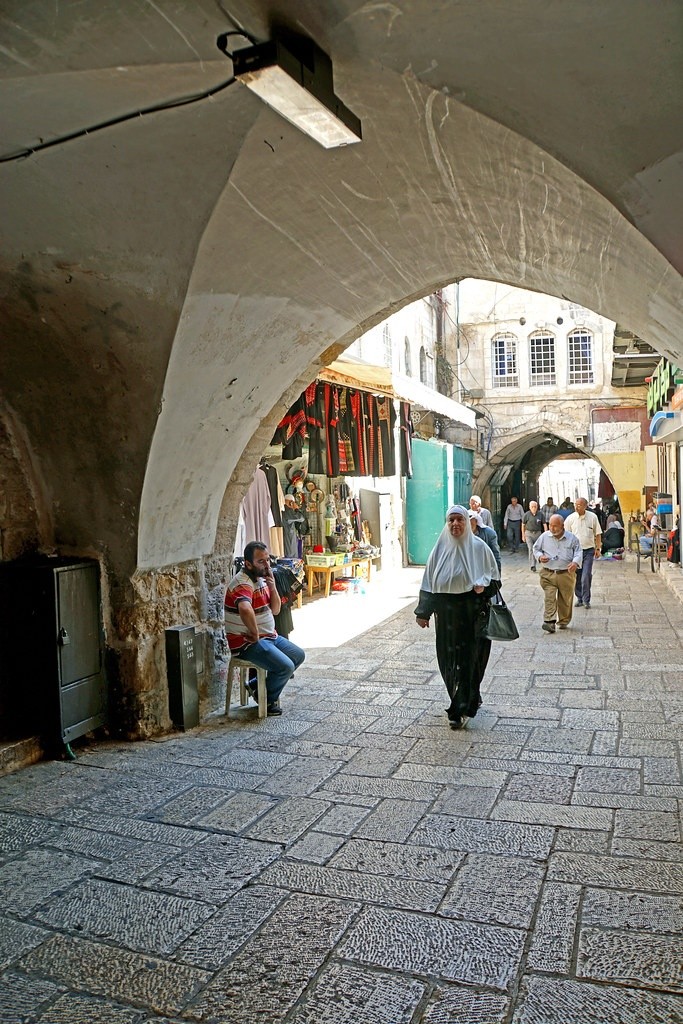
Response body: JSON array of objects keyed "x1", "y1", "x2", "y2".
[{"x1": 305, "y1": 547, "x2": 380, "y2": 568}]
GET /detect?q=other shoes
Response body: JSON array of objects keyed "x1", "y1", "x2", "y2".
[
  {"x1": 531, "y1": 566, "x2": 536, "y2": 571},
  {"x1": 542, "y1": 622, "x2": 556, "y2": 632},
  {"x1": 560, "y1": 625, "x2": 566, "y2": 629},
  {"x1": 448, "y1": 714, "x2": 463, "y2": 727},
  {"x1": 574, "y1": 600, "x2": 582, "y2": 607},
  {"x1": 583, "y1": 602, "x2": 591, "y2": 609}
]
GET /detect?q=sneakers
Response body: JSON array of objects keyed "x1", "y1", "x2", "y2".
[
  {"x1": 252, "y1": 693, "x2": 282, "y2": 715},
  {"x1": 244, "y1": 678, "x2": 258, "y2": 696}
]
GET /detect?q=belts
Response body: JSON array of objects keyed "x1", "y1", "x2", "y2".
[
  {"x1": 544, "y1": 568, "x2": 567, "y2": 573},
  {"x1": 508, "y1": 519, "x2": 520, "y2": 522}
]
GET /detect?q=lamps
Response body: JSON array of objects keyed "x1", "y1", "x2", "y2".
[{"x1": 231, "y1": 24, "x2": 363, "y2": 150}]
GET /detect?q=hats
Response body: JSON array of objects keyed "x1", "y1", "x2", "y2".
[
  {"x1": 467, "y1": 510, "x2": 475, "y2": 520},
  {"x1": 471, "y1": 495, "x2": 481, "y2": 505},
  {"x1": 448, "y1": 507, "x2": 464, "y2": 516}
]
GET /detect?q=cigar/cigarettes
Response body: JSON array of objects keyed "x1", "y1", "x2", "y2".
[{"x1": 269, "y1": 568, "x2": 278, "y2": 571}]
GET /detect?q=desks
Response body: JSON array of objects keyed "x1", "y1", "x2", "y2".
[
  {"x1": 304, "y1": 553, "x2": 381, "y2": 600},
  {"x1": 289, "y1": 578, "x2": 303, "y2": 608}
]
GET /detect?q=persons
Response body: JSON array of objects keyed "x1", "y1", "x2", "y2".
[
  {"x1": 532, "y1": 514, "x2": 583, "y2": 633},
  {"x1": 467, "y1": 509, "x2": 501, "y2": 605},
  {"x1": 414, "y1": 506, "x2": 502, "y2": 728},
  {"x1": 281, "y1": 494, "x2": 310, "y2": 558},
  {"x1": 470, "y1": 496, "x2": 494, "y2": 529},
  {"x1": 224, "y1": 541, "x2": 305, "y2": 716},
  {"x1": 521, "y1": 501, "x2": 548, "y2": 571},
  {"x1": 504, "y1": 497, "x2": 680, "y2": 568},
  {"x1": 563, "y1": 498, "x2": 603, "y2": 610}
]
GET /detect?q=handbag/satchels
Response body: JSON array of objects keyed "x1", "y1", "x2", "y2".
[{"x1": 481, "y1": 581, "x2": 520, "y2": 641}]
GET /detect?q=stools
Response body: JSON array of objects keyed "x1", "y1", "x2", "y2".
[{"x1": 224, "y1": 657, "x2": 268, "y2": 719}]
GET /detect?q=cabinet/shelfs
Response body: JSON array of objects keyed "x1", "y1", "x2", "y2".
[{"x1": 0, "y1": 555, "x2": 108, "y2": 761}]
